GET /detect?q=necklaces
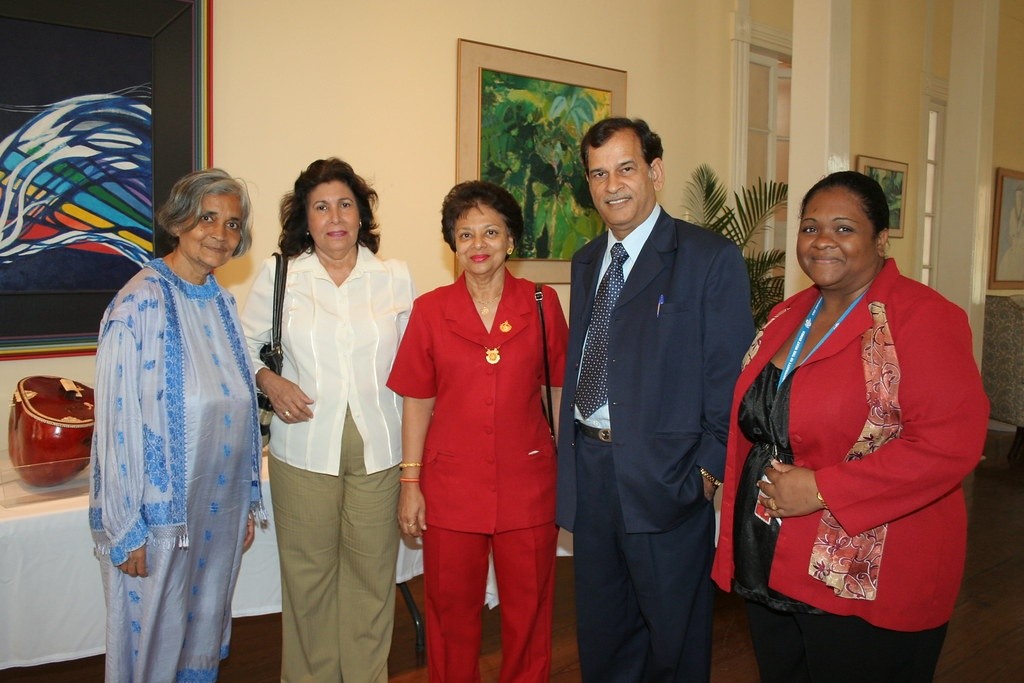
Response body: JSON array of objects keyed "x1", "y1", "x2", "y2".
[{"x1": 472, "y1": 294, "x2": 501, "y2": 316}]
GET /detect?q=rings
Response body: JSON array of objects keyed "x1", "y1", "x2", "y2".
[
  {"x1": 284, "y1": 410, "x2": 290, "y2": 417},
  {"x1": 407, "y1": 522, "x2": 416, "y2": 527},
  {"x1": 770, "y1": 500, "x2": 778, "y2": 510}
]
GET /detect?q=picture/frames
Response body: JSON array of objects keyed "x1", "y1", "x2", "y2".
[
  {"x1": 455, "y1": 37, "x2": 627, "y2": 283},
  {"x1": 0, "y1": 0, "x2": 215, "y2": 360},
  {"x1": 987, "y1": 168, "x2": 1024, "y2": 292},
  {"x1": 855, "y1": 155, "x2": 908, "y2": 239}
]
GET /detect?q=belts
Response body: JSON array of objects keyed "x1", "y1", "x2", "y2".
[{"x1": 575, "y1": 422, "x2": 612, "y2": 443}]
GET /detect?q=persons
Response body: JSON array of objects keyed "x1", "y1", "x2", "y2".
[
  {"x1": 555, "y1": 117, "x2": 756, "y2": 683},
  {"x1": 85, "y1": 167, "x2": 269, "y2": 683},
  {"x1": 384, "y1": 181, "x2": 569, "y2": 683},
  {"x1": 709, "y1": 170, "x2": 991, "y2": 683},
  {"x1": 240, "y1": 158, "x2": 413, "y2": 683}
]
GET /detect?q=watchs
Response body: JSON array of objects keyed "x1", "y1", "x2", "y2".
[
  {"x1": 700, "y1": 467, "x2": 721, "y2": 489},
  {"x1": 817, "y1": 492, "x2": 829, "y2": 510}
]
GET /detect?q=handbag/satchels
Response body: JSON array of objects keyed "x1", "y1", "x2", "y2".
[{"x1": 257, "y1": 340, "x2": 284, "y2": 447}]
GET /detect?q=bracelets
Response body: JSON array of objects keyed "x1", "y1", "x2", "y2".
[
  {"x1": 400, "y1": 478, "x2": 420, "y2": 481},
  {"x1": 399, "y1": 463, "x2": 421, "y2": 467}
]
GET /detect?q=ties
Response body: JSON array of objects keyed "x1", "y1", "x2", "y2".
[{"x1": 575, "y1": 243, "x2": 630, "y2": 421}]
